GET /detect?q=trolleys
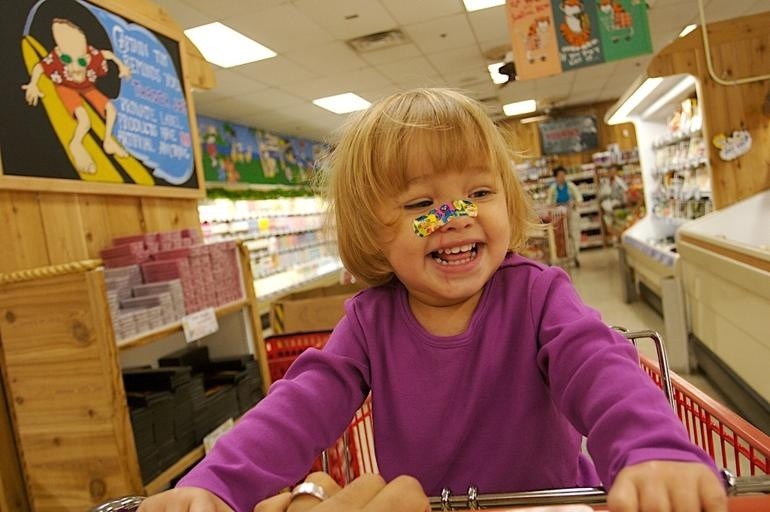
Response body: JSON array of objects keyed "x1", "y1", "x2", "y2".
[
  {"x1": 520, "y1": 202, "x2": 580, "y2": 272},
  {"x1": 91, "y1": 324, "x2": 770, "y2": 510}
]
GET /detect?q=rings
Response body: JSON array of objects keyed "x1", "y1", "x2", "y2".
[{"x1": 285, "y1": 483, "x2": 329, "y2": 502}]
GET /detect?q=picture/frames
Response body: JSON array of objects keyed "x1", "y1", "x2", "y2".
[{"x1": 0, "y1": 1, "x2": 207, "y2": 199}]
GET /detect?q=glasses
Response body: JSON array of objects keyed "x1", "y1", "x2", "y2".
[{"x1": 59, "y1": 53, "x2": 89, "y2": 66}]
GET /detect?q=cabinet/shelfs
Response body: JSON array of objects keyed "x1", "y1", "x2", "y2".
[
  {"x1": 0, "y1": 241, "x2": 272, "y2": 511},
  {"x1": 594, "y1": 157, "x2": 648, "y2": 248},
  {"x1": 541, "y1": 168, "x2": 604, "y2": 248},
  {"x1": 603, "y1": 11, "x2": 770, "y2": 375}
]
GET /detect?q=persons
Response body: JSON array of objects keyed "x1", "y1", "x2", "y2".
[
  {"x1": 547, "y1": 166, "x2": 584, "y2": 269},
  {"x1": 136, "y1": 85, "x2": 726, "y2": 510},
  {"x1": 21, "y1": 18, "x2": 135, "y2": 174},
  {"x1": 243, "y1": 468, "x2": 431, "y2": 512}
]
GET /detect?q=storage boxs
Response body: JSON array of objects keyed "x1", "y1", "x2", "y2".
[{"x1": 269, "y1": 283, "x2": 372, "y2": 335}]
